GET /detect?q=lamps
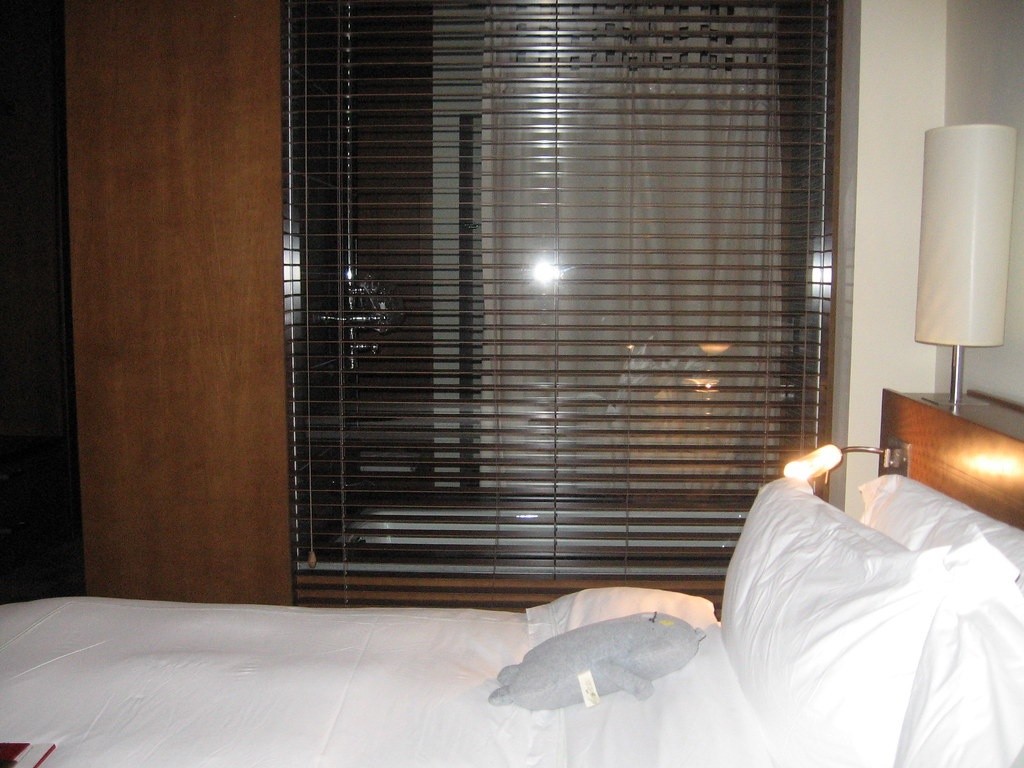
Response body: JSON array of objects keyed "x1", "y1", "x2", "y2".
[
  {"x1": 784, "y1": 433, "x2": 912, "y2": 480},
  {"x1": 914, "y1": 124, "x2": 1017, "y2": 405}
]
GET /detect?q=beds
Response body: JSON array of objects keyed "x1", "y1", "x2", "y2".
[{"x1": 2, "y1": 389, "x2": 1024, "y2": 768}]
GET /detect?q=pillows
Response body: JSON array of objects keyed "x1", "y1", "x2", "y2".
[
  {"x1": 722, "y1": 474, "x2": 1024, "y2": 768},
  {"x1": 526, "y1": 587, "x2": 752, "y2": 768}
]
